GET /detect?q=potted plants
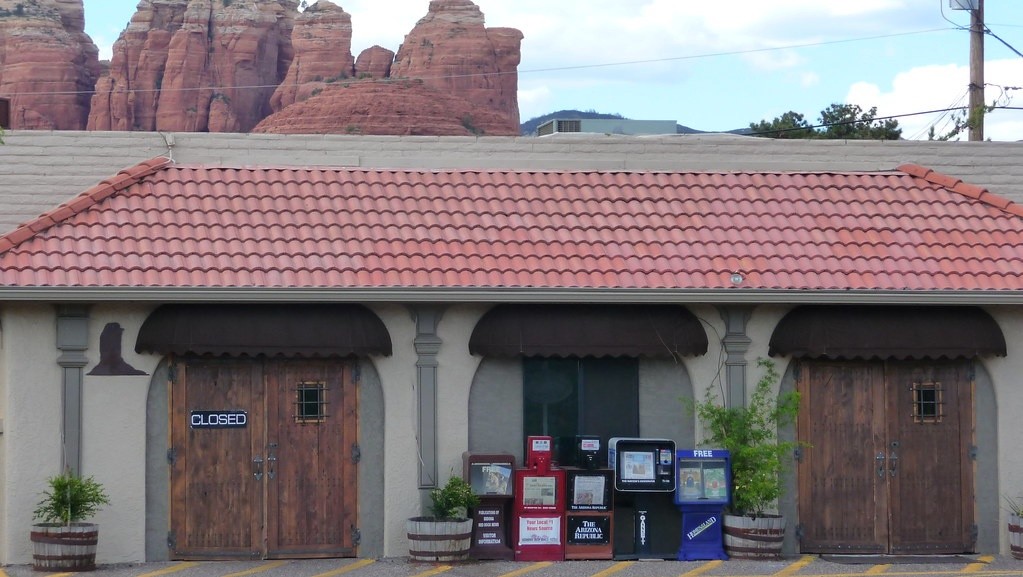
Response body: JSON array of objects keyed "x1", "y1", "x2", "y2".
[
  {"x1": 1000, "y1": 493, "x2": 1023, "y2": 560},
  {"x1": 406, "y1": 468, "x2": 481, "y2": 563},
  {"x1": 678, "y1": 356, "x2": 815, "y2": 559},
  {"x1": 31, "y1": 468, "x2": 112, "y2": 571}
]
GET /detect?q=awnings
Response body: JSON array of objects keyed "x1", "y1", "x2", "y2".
[
  {"x1": 468, "y1": 302, "x2": 708, "y2": 359},
  {"x1": 768, "y1": 304, "x2": 1007, "y2": 361},
  {"x1": 134, "y1": 304, "x2": 392, "y2": 358}
]
